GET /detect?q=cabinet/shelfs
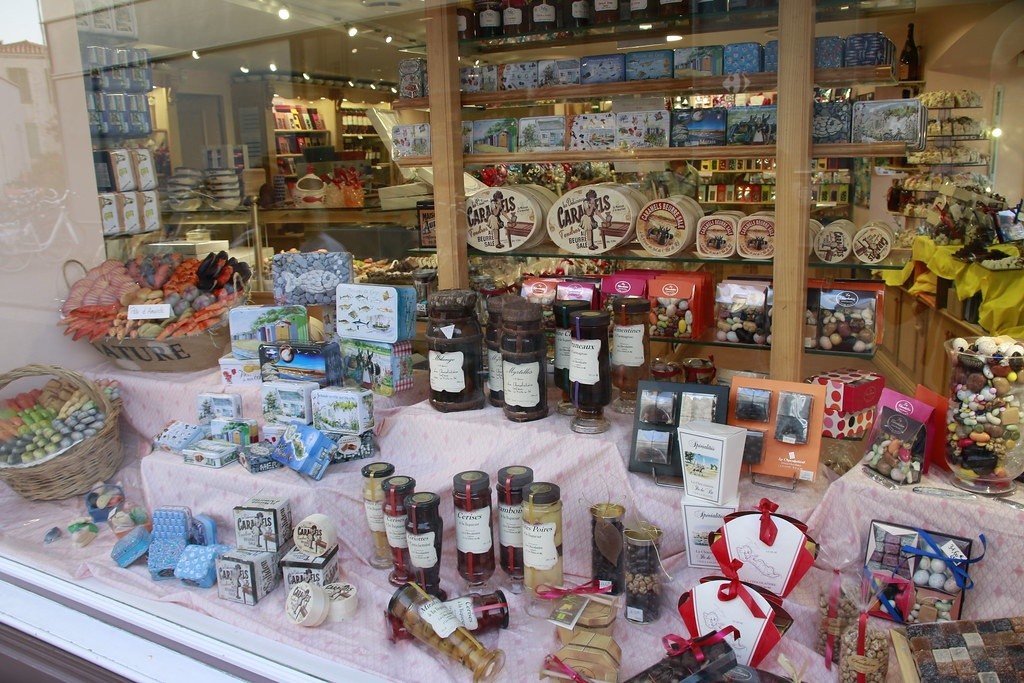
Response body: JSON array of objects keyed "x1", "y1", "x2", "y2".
[
  {"x1": 395, "y1": 0, "x2": 922, "y2": 386},
  {"x1": 908, "y1": 105, "x2": 992, "y2": 166},
  {"x1": 231, "y1": 80, "x2": 396, "y2": 182}
]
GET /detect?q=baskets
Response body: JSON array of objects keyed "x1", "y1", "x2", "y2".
[{"x1": 0, "y1": 364, "x2": 123, "y2": 501}]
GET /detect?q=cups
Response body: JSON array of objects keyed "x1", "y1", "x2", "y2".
[{"x1": 942, "y1": 335, "x2": 1023, "y2": 494}]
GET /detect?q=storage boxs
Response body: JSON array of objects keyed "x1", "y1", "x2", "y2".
[{"x1": 75, "y1": 1, "x2": 973, "y2": 627}]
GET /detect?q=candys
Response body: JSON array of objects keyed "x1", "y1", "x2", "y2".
[{"x1": 593, "y1": 292, "x2": 1024, "y2": 683}]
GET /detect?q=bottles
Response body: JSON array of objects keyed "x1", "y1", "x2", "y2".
[
  {"x1": 383, "y1": 590, "x2": 509, "y2": 645},
  {"x1": 426, "y1": 288, "x2": 650, "y2": 433},
  {"x1": 361, "y1": 465, "x2": 564, "y2": 601},
  {"x1": 623, "y1": 525, "x2": 664, "y2": 625},
  {"x1": 387, "y1": 582, "x2": 506, "y2": 683},
  {"x1": 590, "y1": 503, "x2": 626, "y2": 596}
]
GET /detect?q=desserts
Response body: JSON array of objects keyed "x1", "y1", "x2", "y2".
[{"x1": 898, "y1": 89, "x2": 980, "y2": 250}]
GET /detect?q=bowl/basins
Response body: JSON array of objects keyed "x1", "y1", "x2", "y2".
[{"x1": 167, "y1": 166, "x2": 241, "y2": 209}]
